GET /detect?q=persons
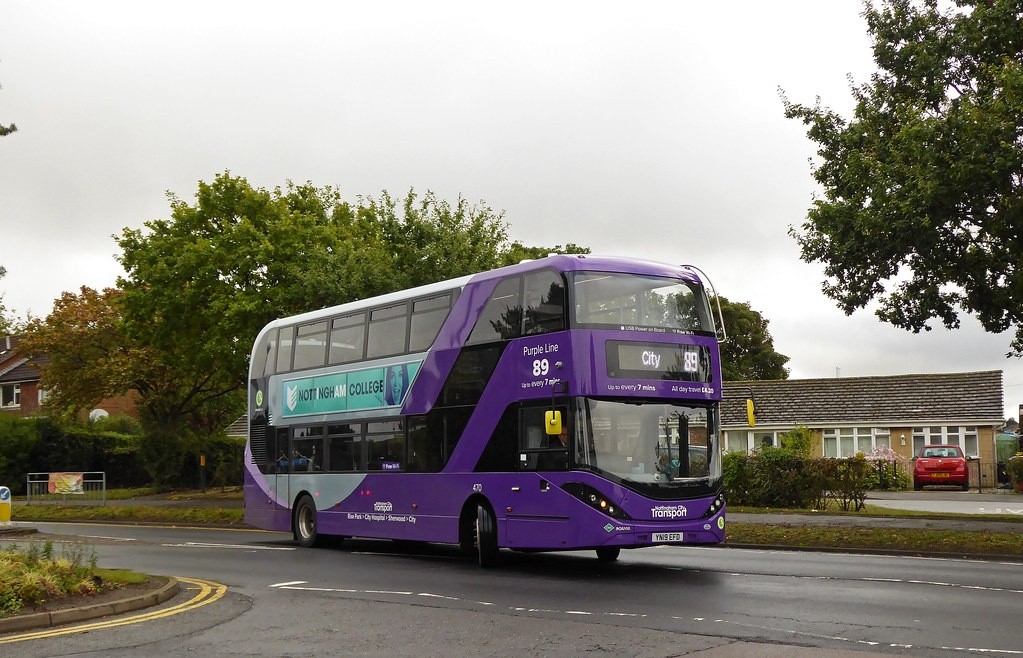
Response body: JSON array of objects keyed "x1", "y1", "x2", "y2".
[
  {"x1": 386, "y1": 365, "x2": 409, "y2": 407},
  {"x1": 551, "y1": 426, "x2": 582, "y2": 461}
]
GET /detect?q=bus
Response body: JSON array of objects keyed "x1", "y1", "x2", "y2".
[{"x1": 241, "y1": 250, "x2": 759, "y2": 566}]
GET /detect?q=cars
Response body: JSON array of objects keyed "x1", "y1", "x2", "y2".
[{"x1": 912, "y1": 443, "x2": 972, "y2": 491}]
